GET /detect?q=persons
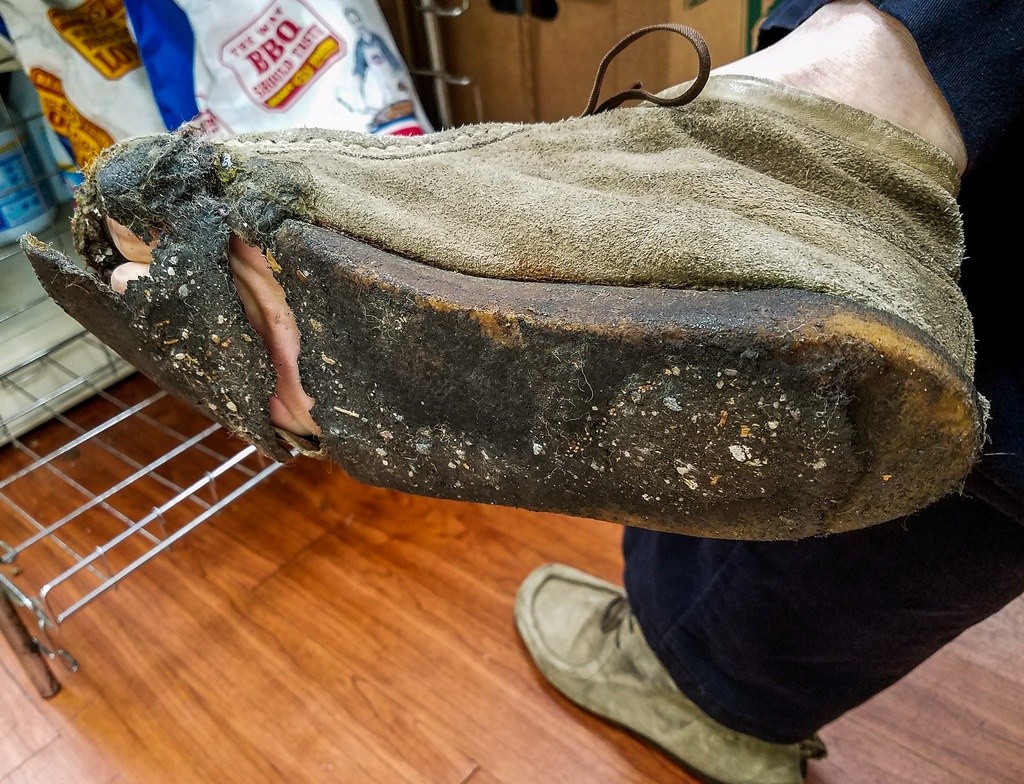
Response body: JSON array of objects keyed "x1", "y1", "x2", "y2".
[{"x1": 16, "y1": 0, "x2": 1024, "y2": 784}]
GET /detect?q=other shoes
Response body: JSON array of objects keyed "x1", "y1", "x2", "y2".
[
  {"x1": 511, "y1": 561, "x2": 830, "y2": 783},
  {"x1": 15, "y1": 30, "x2": 994, "y2": 541}
]
